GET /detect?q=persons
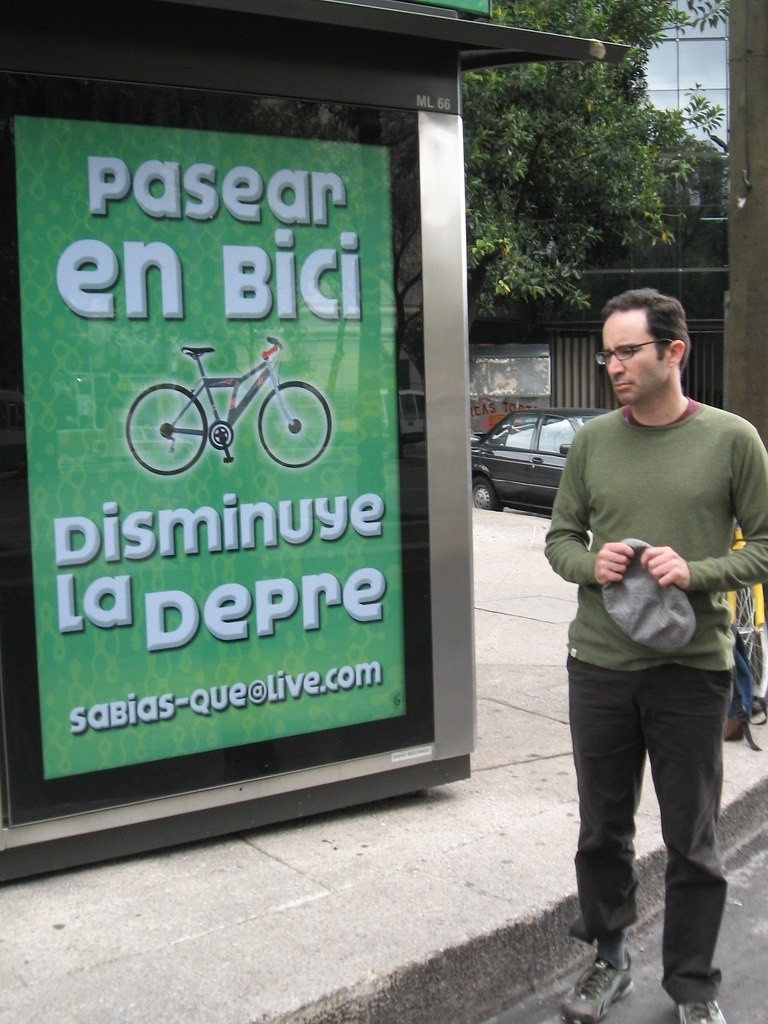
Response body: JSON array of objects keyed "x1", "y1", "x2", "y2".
[{"x1": 543, "y1": 287, "x2": 768, "y2": 1024}]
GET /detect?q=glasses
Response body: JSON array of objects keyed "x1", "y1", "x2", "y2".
[{"x1": 594, "y1": 339, "x2": 674, "y2": 365}]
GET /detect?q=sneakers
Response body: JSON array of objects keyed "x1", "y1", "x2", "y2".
[
  {"x1": 561, "y1": 950, "x2": 634, "y2": 1024},
  {"x1": 676, "y1": 998, "x2": 726, "y2": 1024}
]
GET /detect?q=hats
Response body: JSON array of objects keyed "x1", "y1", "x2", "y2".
[{"x1": 601, "y1": 538, "x2": 695, "y2": 654}]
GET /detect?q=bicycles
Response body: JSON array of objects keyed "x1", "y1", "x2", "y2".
[
  {"x1": 124, "y1": 337, "x2": 334, "y2": 476},
  {"x1": 726, "y1": 523, "x2": 767, "y2": 704}
]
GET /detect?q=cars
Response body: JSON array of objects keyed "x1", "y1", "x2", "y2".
[{"x1": 471, "y1": 406, "x2": 613, "y2": 516}]
